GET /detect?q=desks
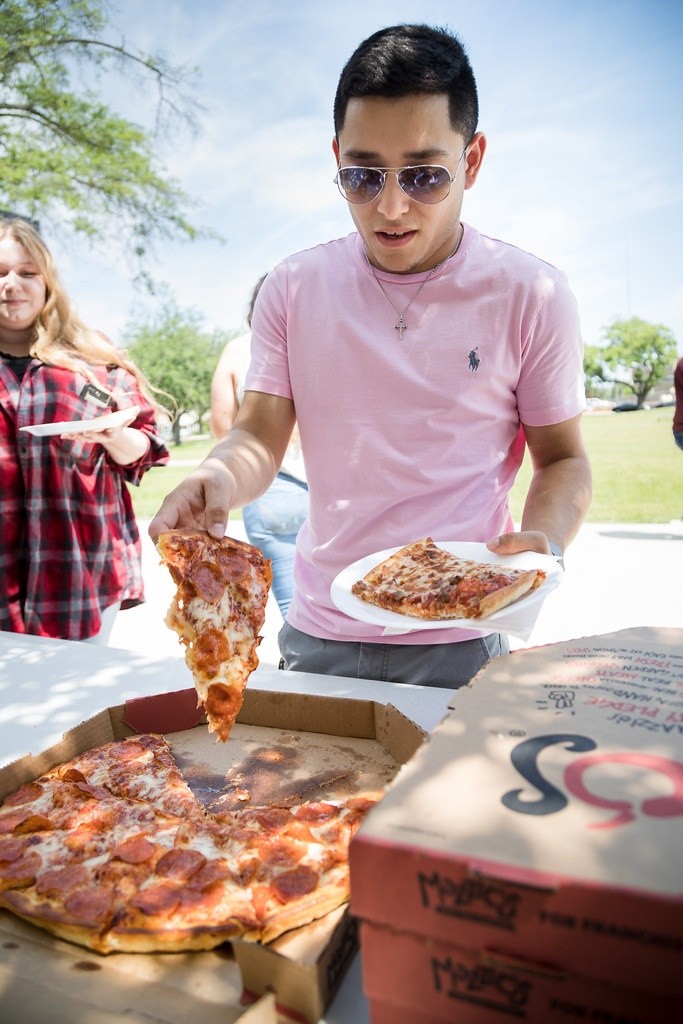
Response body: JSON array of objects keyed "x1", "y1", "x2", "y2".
[{"x1": 0, "y1": 635, "x2": 458, "y2": 1024}]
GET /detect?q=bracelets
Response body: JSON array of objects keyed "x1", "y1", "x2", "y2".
[{"x1": 549, "y1": 542, "x2": 565, "y2": 573}]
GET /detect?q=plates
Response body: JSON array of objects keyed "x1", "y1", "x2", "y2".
[
  {"x1": 329, "y1": 541, "x2": 563, "y2": 628},
  {"x1": 19, "y1": 406, "x2": 140, "y2": 436}
]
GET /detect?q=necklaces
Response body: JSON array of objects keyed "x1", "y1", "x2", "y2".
[{"x1": 362, "y1": 223, "x2": 460, "y2": 339}]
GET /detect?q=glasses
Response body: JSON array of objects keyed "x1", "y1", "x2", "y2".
[
  {"x1": 334, "y1": 150, "x2": 466, "y2": 206},
  {"x1": 0, "y1": 211, "x2": 39, "y2": 230}
]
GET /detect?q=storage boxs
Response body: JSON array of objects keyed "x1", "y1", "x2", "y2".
[{"x1": 347, "y1": 625, "x2": 683, "y2": 1024}]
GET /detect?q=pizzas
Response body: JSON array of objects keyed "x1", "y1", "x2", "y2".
[
  {"x1": 0, "y1": 734, "x2": 385, "y2": 955},
  {"x1": 156, "y1": 529, "x2": 272, "y2": 743},
  {"x1": 352, "y1": 536, "x2": 547, "y2": 620}
]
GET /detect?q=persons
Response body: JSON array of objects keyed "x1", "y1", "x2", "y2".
[
  {"x1": 149, "y1": 23, "x2": 594, "y2": 690},
  {"x1": 212, "y1": 273, "x2": 310, "y2": 621},
  {"x1": 0, "y1": 210, "x2": 180, "y2": 648},
  {"x1": 672, "y1": 357, "x2": 683, "y2": 451}
]
